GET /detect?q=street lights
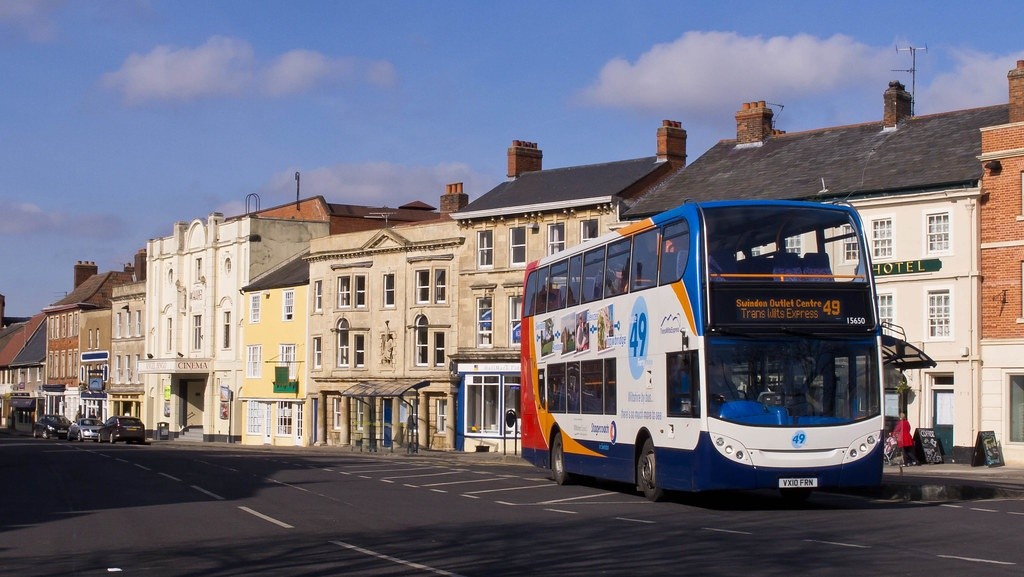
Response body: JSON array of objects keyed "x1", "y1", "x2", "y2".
[{"x1": 221, "y1": 385, "x2": 234, "y2": 444}]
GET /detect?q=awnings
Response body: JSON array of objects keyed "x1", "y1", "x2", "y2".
[
  {"x1": 9, "y1": 399, "x2": 36, "y2": 408},
  {"x1": 342, "y1": 382, "x2": 422, "y2": 408}
]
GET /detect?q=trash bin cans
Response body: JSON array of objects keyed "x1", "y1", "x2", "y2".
[{"x1": 156, "y1": 422, "x2": 169, "y2": 440}]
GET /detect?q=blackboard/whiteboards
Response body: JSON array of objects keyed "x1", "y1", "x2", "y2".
[
  {"x1": 971, "y1": 431, "x2": 1002, "y2": 466},
  {"x1": 912, "y1": 427, "x2": 943, "y2": 463}
]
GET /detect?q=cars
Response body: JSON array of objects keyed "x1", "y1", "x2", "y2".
[
  {"x1": 32, "y1": 414, "x2": 72, "y2": 440},
  {"x1": 97, "y1": 415, "x2": 146, "y2": 444},
  {"x1": 66, "y1": 418, "x2": 105, "y2": 442}
]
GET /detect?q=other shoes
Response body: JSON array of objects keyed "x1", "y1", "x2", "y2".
[
  {"x1": 916, "y1": 461, "x2": 921, "y2": 465},
  {"x1": 901, "y1": 463, "x2": 909, "y2": 467}
]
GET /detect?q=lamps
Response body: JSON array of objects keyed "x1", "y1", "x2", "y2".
[
  {"x1": 527, "y1": 222, "x2": 539, "y2": 229},
  {"x1": 148, "y1": 354, "x2": 152, "y2": 358},
  {"x1": 984, "y1": 160, "x2": 1000, "y2": 170}
]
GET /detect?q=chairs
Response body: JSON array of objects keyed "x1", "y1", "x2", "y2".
[
  {"x1": 558, "y1": 383, "x2": 616, "y2": 414},
  {"x1": 558, "y1": 245, "x2": 835, "y2": 303}
]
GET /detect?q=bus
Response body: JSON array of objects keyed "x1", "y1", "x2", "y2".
[{"x1": 520, "y1": 196, "x2": 908, "y2": 506}]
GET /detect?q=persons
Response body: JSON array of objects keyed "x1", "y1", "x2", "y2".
[
  {"x1": 380, "y1": 324, "x2": 395, "y2": 362},
  {"x1": 889, "y1": 413, "x2": 916, "y2": 466},
  {"x1": 576, "y1": 314, "x2": 588, "y2": 351},
  {"x1": 605, "y1": 263, "x2": 628, "y2": 296},
  {"x1": 710, "y1": 360, "x2": 738, "y2": 400}
]
